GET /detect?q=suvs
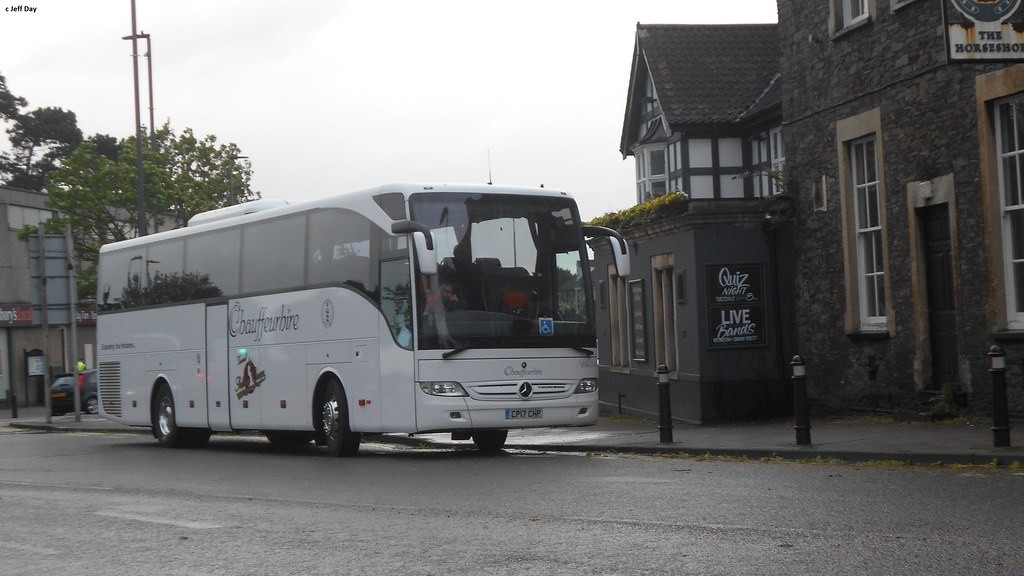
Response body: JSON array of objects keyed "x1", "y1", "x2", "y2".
[{"x1": 50, "y1": 368, "x2": 100, "y2": 415}]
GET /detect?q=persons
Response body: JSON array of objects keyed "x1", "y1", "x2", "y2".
[
  {"x1": 78, "y1": 358, "x2": 85, "y2": 371},
  {"x1": 419, "y1": 273, "x2": 457, "y2": 314}
]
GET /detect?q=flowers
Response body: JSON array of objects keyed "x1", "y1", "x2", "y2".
[{"x1": 588, "y1": 188, "x2": 689, "y2": 228}]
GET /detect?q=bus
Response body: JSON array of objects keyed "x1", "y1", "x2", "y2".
[{"x1": 94, "y1": 180, "x2": 632, "y2": 458}]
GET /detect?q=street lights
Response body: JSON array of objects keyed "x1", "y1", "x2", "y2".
[{"x1": 121, "y1": 31, "x2": 159, "y2": 235}]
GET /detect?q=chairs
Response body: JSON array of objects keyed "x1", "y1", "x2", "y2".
[
  {"x1": 439, "y1": 257, "x2": 564, "y2": 321},
  {"x1": 191, "y1": 286, "x2": 222, "y2": 300},
  {"x1": 341, "y1": 255, "x2": 371, "y2": 292},
  {"x1": 145, "y1": 292, "x2": 175, "y2": 305}
]
